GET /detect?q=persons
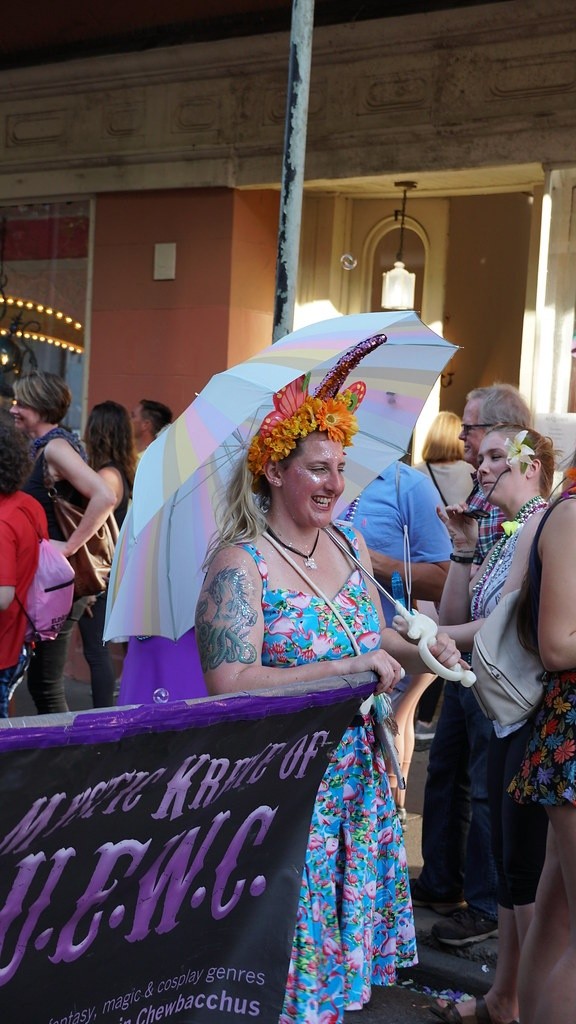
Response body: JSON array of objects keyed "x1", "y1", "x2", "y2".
[
  {"x1": 194, "y1": 398, "x2": 470, "y2": 1024},
  {"x1": 392, "y1": 672, "x2": 437, "y2": 832},
  {"x1": 0, "y1": 420, "x2": 49, "y2": 719},
  {"x1": 334, "y1": 456, "x2": 454, "y2": 697},
  {"x1": 500, "y1": 449, "x2": 576, "y2": 1024},
  {"x1": 413, "y1": 411, "x2": 476, "y2": 740},
  {"x1": 77, "y1": 401, "x2": 137, "y2": 708},
  {"x1": 130, "y1": 399, "x2": 173, "y2": 458},
  {"x1": 407, "y1": 383, "x2": 529, "y2": 945},
  {"x1": 391, "y1": 423, "x2": 555, "y2": 1024},
  {"x1": 14, "y1": 370, "x2": 118, "y2": 717}
]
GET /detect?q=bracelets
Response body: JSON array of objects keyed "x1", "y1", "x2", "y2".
[{"x1": 450, "y1": 553, "x2": 474, "y2": 563}]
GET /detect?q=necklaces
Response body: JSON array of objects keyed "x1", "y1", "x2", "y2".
[
  {"x1": 468, "y1": 495, "x2": 548, "y2": 663},
  {"x1": 267, "y1": 527, "x2": 320, "y2": 570},
  {"x1": 345, "y1": 494, "x2": 361, "y2": 522}
]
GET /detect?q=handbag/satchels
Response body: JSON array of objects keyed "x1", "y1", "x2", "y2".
[
  {"x1": 472, "y1": 590, "x2": 546, "y2": 727},
  {"x1": 43, "y1": 459, "x2": 121, "y2": 597},
  {"x1": 399, "y1": 813, "x2": 425, "y2": 878}
]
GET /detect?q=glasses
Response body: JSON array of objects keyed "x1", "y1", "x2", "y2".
[
  {"x1": 461, "y1": 423, "x2": 497, "y2": 436},
  {"x1": 461, "y1": 467, "x2": 513, "y2": 521}
]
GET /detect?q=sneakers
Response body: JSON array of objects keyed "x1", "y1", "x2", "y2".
[
  {"x1": 432, "y1": 907, "x2": 498, "y2": 946},
  {"x1": 408, "y1": 877, "x2": 468, "y2": 913}
]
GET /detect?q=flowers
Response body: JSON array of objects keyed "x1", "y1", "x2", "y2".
[
  {"x1": 504, "y1": 429, "x2": 537, "y2": 473},
  {"x1": 247, "y1": 398, "x2": 359, "y2": 476},
  {"x1": 501, "y1": 519, "x2": 520, "y2": 536}
]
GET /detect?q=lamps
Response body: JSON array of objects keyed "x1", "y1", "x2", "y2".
[{"x1": 380, "y1": 182, "x2": 416, "y2": 310}]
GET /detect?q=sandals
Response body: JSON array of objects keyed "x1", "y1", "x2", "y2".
[{"x1": 427, "y1": 992, "x2": 520, "y2": 1024}]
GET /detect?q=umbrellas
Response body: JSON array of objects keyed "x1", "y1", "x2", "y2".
[{"x1": 103, "y1": 311, "x2": 465, "y2": 689}]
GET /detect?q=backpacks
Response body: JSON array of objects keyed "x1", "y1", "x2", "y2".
[{"x1": 25, "y1": 539, "x2": 76, "y2": 641}]
